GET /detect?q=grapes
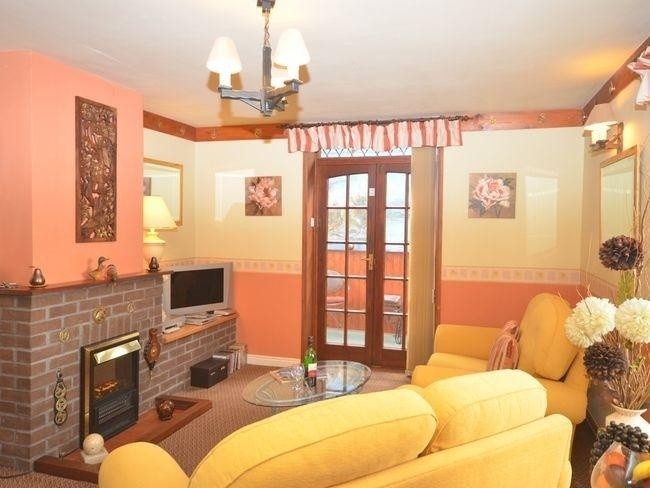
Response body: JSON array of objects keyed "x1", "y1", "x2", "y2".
[{"x1": 590, "y1": 420, "x2": 650, "y2": 465}]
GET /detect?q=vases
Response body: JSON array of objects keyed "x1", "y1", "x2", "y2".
[{"x1": 604, "y1": 400, "x2": 649, "y2": 437}]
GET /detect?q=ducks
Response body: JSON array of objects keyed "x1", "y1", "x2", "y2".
[{"x1": 87, "y1": 256, "x2": 110, "y2": 279}]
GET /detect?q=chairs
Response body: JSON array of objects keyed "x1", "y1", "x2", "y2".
[
  {"x1": 411, "y1": 294, "x2": 589, "y2": 461},
  {"x1": 326, "y1": 270, "x2": 345, "y2": 331}
]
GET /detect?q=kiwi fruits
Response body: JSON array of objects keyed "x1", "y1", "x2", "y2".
[{"x1": 604, "y1": 465, "x2": 626, "y2": 488}]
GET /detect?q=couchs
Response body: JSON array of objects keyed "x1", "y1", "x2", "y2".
[{"x1": 98, "y1": 384, "x2": 572, "y2": 488}]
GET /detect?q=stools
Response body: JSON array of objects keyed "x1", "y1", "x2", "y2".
[{"x1": 384, "y1": 294, "x2": 402, "y2": 344}]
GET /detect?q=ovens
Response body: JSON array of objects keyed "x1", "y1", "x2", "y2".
[{"x1": 80, "y1": 330, "x2": 142, "y2": 449}]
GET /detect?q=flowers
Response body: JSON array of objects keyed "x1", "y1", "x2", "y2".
[{"x1": 557, "y1": 194, "x2": 650, "y2": 410}]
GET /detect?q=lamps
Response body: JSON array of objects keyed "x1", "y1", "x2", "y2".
[
  {"x1": 206, "y1": 0, "x2": 311, "y2": 118},
  {"x1": 143, "y1": 195, "x2": 177, "y2": 264},
  {"x1": 582, "y1": 103, "x2": 624, "y2": 155}
]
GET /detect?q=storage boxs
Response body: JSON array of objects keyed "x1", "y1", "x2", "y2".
[{"x1": 190, "y1": 358, "x2": 228, "y2": 389}]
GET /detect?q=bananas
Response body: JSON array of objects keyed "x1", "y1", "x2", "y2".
[{"x1": 631, "y1": 460, "x2": 650, "y2": 485}]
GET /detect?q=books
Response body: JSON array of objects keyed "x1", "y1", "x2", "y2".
[
  {"x1": 212, "y1": 342, "x2": 246, "y2": 374},
  {"x1": 270, "y1": 369, "x2": 327, "y2": 384}
]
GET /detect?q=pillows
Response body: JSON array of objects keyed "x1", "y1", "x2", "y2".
[
  {"x1": 502, "y1": 320, "x2": 520, "y2": 342},
  {"x1": 486, "y1": 331, "x2": 519, "y2": 371}
]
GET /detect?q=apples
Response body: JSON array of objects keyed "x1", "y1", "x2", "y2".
[{"x1": 605, "y1": 452, "x2": 625, "y2": 468}]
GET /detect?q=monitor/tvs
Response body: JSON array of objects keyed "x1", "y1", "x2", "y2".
[{"x1": 163, "y1": 262, "x2": 233, "y2": 315}]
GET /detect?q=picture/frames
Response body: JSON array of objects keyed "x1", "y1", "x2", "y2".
[{"x1": 75, "y1": 96, "x2": 117, "y2": 242}]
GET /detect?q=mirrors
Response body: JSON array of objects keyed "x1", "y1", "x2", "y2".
[
  {"x1": 143, "y1": 158, "x2": 183, "y2": 226},
  {"x1": 599, "y1": 145, "x2": 639, "y2": 254}
]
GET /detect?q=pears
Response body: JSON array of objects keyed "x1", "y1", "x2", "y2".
[
  {"x1": 29, "y1": 265, "x2": 45, "y2": 286},
  {"x1": 148, "y1": 256, "x2": 159, "y2": 269}
]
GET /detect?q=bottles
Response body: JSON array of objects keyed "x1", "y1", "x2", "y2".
[{"x1": 303, "y1": 335, "x2": 318, "y2": 386}]
